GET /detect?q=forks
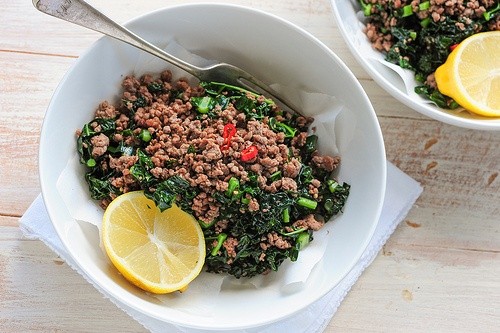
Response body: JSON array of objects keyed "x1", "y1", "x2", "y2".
[{"x1": 33, "y1": 0, "x2": 307, "y2": 121}]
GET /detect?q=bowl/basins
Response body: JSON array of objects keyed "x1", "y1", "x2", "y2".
[
  {"x1": 333, "y1": 0, "x2": 500, "y2": 131},
  {"x1": 37, "y1": 3, "x2": 387, "y2": 330}
]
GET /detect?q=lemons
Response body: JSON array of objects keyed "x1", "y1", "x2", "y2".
[
  {"x1": 435, "y1": 31, "x2": 500, "y2": 117},
  {"x1": 102, "y1": 190, "x2": 206, "y2": 293}
]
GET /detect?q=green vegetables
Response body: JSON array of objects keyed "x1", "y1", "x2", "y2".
[
  {"x1": 75, "y1": 68, "x2": 351, "y2": 279},
  {"x1": 348, "y1": 1, "x2": 500, "y2": 109}
]
